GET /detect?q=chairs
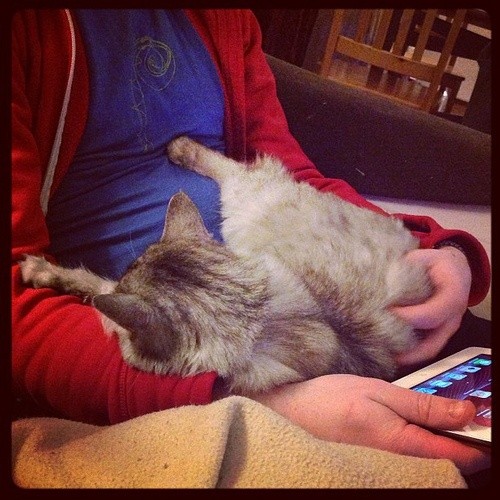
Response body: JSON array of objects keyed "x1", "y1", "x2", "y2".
[{"x1": 315, "y1": 9, "x2": 470, "y2": 116}]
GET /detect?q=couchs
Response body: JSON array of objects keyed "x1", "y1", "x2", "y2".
[{"x1": 260, "y1": 48, "x2": 490, "y2": 215}]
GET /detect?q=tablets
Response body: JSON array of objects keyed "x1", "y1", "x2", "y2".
[{"x1": 392, "y1": 346, "x2": 492, "y2": 443}]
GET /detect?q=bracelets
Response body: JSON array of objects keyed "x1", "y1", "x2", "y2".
[{"x1": 433, "y1": 239, "x2": 469, "y2": 258}]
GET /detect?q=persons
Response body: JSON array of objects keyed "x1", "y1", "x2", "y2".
[{"x1": 7, "y1": 6, "x2": 491, "y2": 478}]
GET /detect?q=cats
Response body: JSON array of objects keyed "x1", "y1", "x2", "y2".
[{"x1": 18, "y1": 135, "x2": 432, "y2": 394}]
